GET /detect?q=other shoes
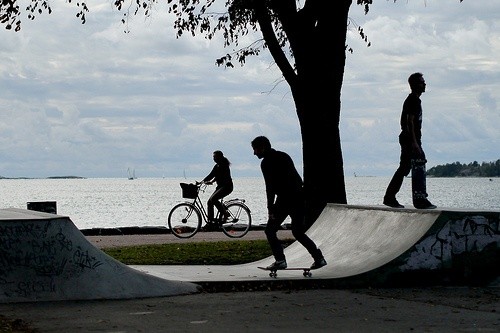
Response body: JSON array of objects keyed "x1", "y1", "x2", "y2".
[
  {"x1": 222, "y1": 211, "x2": 230, "y2": 221},
  {"x1": 416, "y1": 201, "x2": 437, "y2": 209},
  {"x1": 383, "y1": 200, "x2": 404, "y2": 208}
]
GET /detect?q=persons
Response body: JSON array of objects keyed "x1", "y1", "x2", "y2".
[
  {"x1": 199, "y1": 151, "x2": 233, "y2": 228},
  {"x1": 251, "y1": 136, "x2": 327, "y2": 270},
  {"x1": 383, "y1": 72, "x2": 437, "y2": 208}
]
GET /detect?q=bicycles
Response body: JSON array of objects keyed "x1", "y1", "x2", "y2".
[{"x1": 168, "y1": 181, "x2": 252, "y2": 239}]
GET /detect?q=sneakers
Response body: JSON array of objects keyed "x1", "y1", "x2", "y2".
[
  {"x1": 267, "y1": 260, "x2": 287, "y2": 269},
  {"x1": 310, "y1": 259, "x2": 326, "y2": 269}
]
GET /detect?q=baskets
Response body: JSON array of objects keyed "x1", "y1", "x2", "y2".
[{"x1": 183, "y1": 187, "x2": 197, "y2": 198}]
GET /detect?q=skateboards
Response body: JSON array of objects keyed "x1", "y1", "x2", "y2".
[
  {"x1": 256, "y1": 266, "x2": 322, "y2": 278},
  {"x1": 411, "y1": 152, "x2": 429, "y2": 210}
]
